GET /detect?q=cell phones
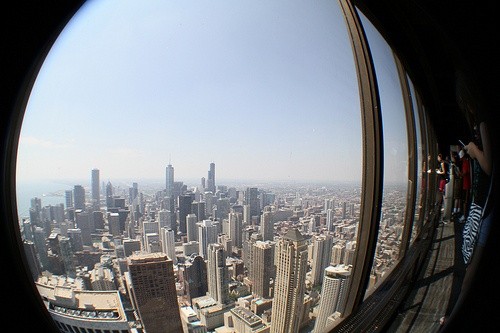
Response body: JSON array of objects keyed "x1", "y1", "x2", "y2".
[{"x1": 459, "y1": 138, "x2": 468, "y2": 147}]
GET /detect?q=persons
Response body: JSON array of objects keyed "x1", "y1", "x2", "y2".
[
  {"x1": 436, "y1": 68, "x2": 500, "y2": 333},
  {"x1": 419, "y1": 152, "x2": 432, "y2": 211}
]
